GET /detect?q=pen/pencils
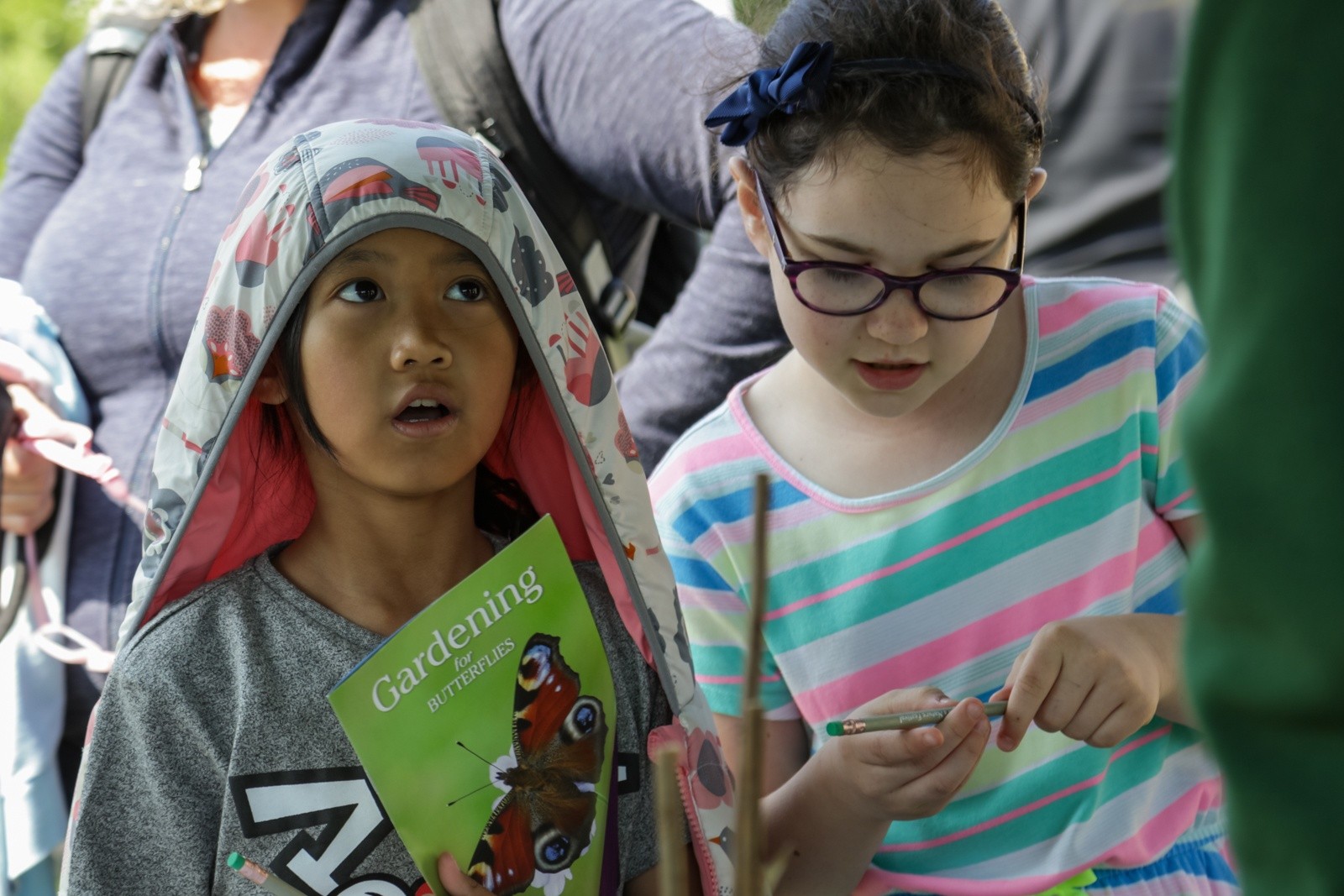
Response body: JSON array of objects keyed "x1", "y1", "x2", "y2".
[
  {"x1": 226, "y1": 852, "x2": 307, "y2": 896},
  {"x1": 826, "y1": 700, "x2": 1008, "y2": 736}
]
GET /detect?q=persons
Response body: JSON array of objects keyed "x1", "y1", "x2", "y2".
[
  {"x1": 0, "y1": 0, "x2": 1190, "y2": 896},
  {"x1": 641, "y1": 0, "x2": 1241, "y2": 896}
]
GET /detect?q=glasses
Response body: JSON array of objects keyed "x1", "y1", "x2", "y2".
[{"x1": 753, "y1": 168, "x2": 1026, "y2": 320}]
